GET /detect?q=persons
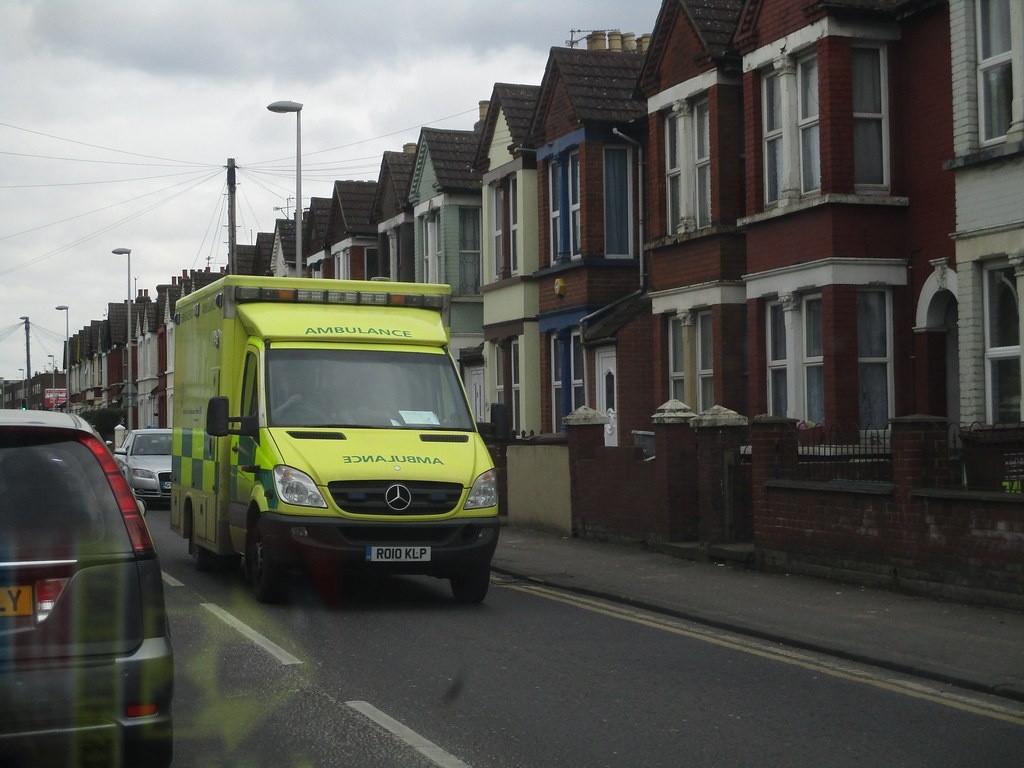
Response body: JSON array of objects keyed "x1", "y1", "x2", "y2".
[{"x1": 269, "y1": 394, "x2": 305, "y2": 422}]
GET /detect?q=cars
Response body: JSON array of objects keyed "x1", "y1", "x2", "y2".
[{"x1": 114, "y1": 429, "x2": 173, "y2": 501}]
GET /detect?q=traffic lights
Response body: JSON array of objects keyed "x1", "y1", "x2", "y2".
[{"x1": 21, "y1": 398, "x2": 28, "y2": 410}]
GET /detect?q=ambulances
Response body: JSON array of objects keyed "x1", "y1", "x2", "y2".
[{"x1": 170, "y1": 274, "x2": 508, "y2": 605}]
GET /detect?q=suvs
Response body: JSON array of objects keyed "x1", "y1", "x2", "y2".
[{"x1": 0, "y1": 408, "x2": 174, "y2": 768}]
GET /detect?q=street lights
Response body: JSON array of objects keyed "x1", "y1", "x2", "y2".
[
  {"x1": 55, "y1": 305, "x2": 70, "y2": 412},
  {"x1": 48, "y1": 355, "x2": 56, "y2": 411},
  {"x1": 19, "y1": 315, "x2": 34, "y2": 411},
  {"x1": 112, "y1": 248, "x2": 134, "y2": 430},
  {"x1": 267, "y1": 100, "x2": 303, "y2": 279},
  {"x1": 19, "y1": 368, "x2": 24, "y2": 398}
]
